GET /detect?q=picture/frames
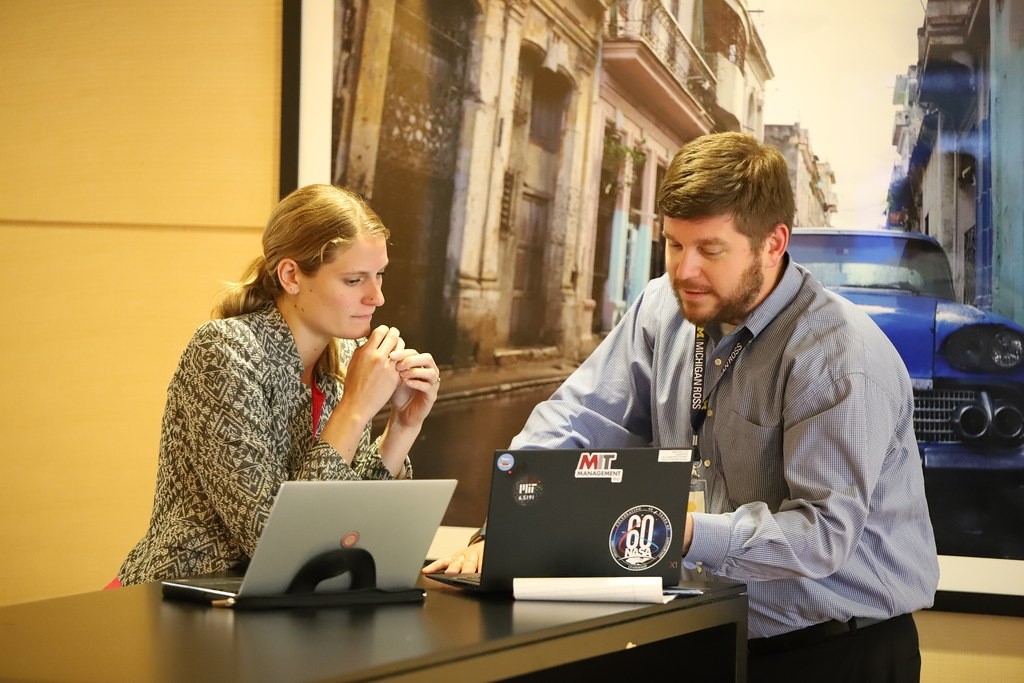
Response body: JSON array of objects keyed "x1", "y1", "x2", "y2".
[{"x1": 280, "y1": 0, "x2": 1024, "y2": 617}]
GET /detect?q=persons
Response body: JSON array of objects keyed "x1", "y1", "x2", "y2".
[
  {"x1": 423, "y1": 131, "x2": 940, "y2": 683},
  {"x1": 102, "y1": 184, "x2": 440, "y2": 593}
]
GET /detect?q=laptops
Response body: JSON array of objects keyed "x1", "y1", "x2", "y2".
[
  {"x1": 425, "y1": 447, "x2": 694, "y2": 588},
  {"x1": 158, "y1": 479, "x2": 459, "y2": 604}
]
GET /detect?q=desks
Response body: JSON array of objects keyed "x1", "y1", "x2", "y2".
[{"x1": 0, "y1": 564, "x2": 750, "y2": 683}]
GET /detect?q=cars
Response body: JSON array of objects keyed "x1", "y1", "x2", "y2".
[{"x1": 778, "y1": 224, "x2": 1024, "y2": 483}]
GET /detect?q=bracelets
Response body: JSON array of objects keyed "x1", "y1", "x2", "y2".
[{"x1": 466, "y1": 516, "x2": 487, "y2": 548}]
